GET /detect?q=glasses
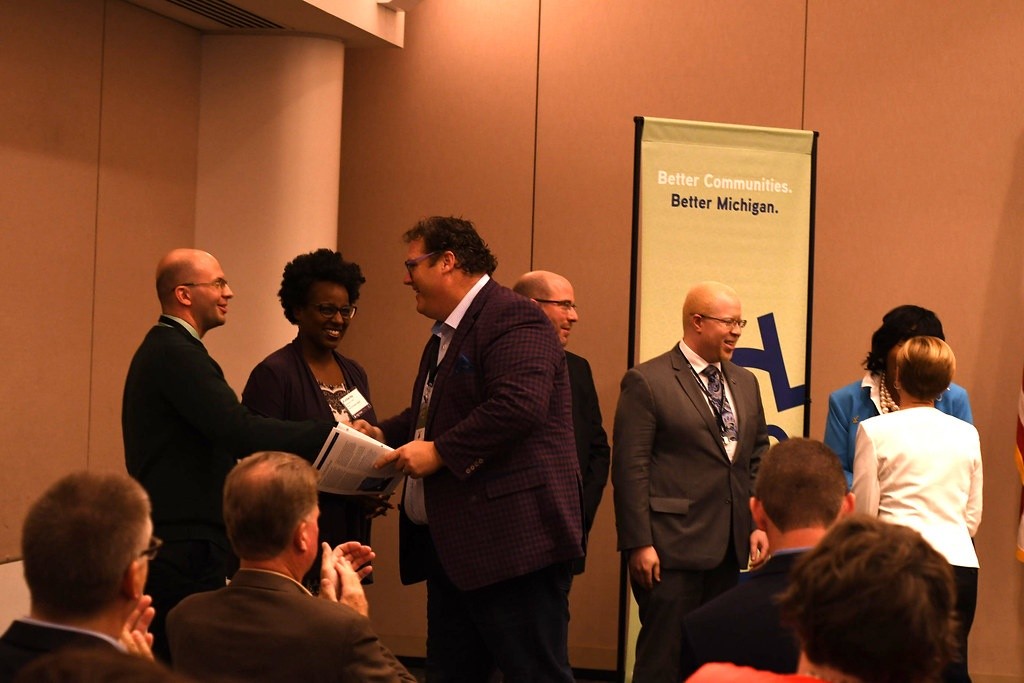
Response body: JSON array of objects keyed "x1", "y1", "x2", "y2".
[
  {"x1": 404, "y1": 249, "x2": 465, "y2": 279},
  {"x1": 171, "y1": 276, "x2": 228, "y2": 295},
  {"x1": 122, "y1": 536, "x2": 163, "y2": 582},
  {"x1": 534, "y1": 298, "x2": 577, "y2": 313},
  {"x1": 310, "y1": 301, "x2": 357, "y2": 319},
  {"x1": 689, "y1": 312, "x2": 747, "y2": 328}
]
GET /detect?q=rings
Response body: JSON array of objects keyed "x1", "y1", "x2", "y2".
[{"x1": 403, "y1": 469, "x2": 410, "y2": 475}]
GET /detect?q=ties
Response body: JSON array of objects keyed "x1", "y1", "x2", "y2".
[{"x1": 702, "y1": 364, "x2": 738, "y2": 442}]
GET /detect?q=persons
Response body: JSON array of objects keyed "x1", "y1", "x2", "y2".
[
  {"x1": 0, "y1": 217, "x2": 610, "y2": 683},
  {"x1": 611, "y1": 278, "x2": 983, "y2": 683}
]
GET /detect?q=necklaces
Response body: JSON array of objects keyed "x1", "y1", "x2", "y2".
[
  {"x1": 880, "y1": 372, "x2": 900, "y2": 414},
  {"x1": 798, "y1": 668, "x2": 849, "y2": 683}
]
